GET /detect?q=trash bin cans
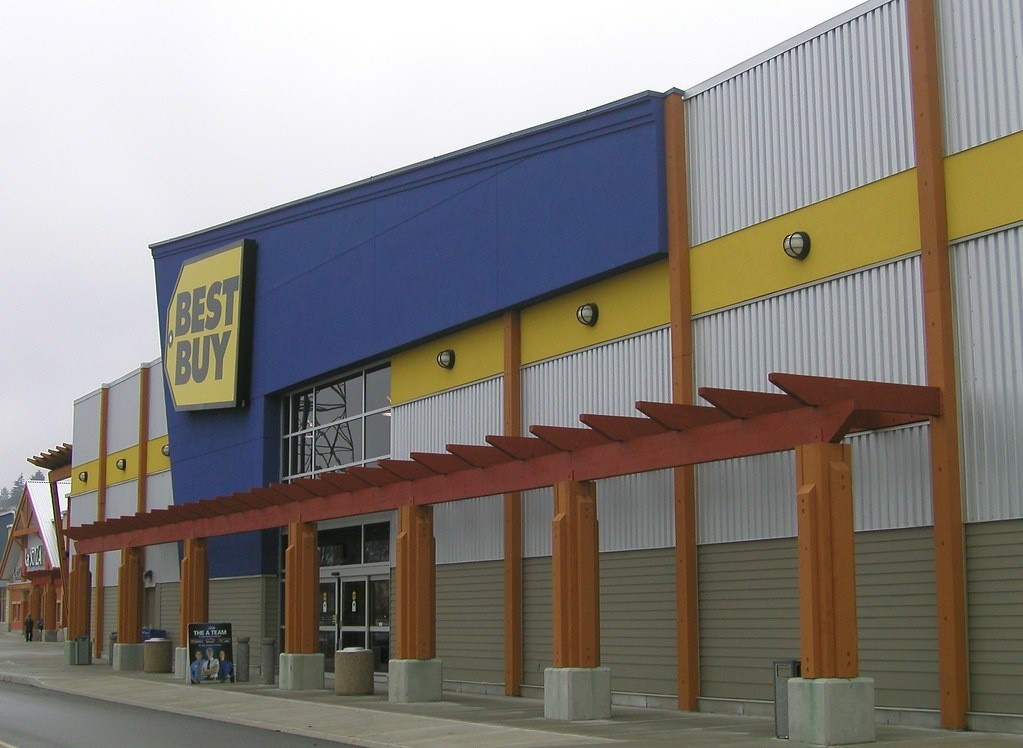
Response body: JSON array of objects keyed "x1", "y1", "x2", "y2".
[{"x1": 65, "y1": 636, "x2": 92, "y2": 664}]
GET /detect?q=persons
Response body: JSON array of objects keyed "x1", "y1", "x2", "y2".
[
  {"x1": 190, "y1": 650, "x2": 208, "y2": 684},
  {"x1": 201, "y1": 648, "x2": 219, "y2": 679},
  {"x1": 217, "y1": 651, "x2": 234, "y2": 681},
  {"x1": 37, "y1": 616, "x2": 43, "y2": 641},
  {"x1": 24, "y1": 614, "x2": 33, "y2": 642}
]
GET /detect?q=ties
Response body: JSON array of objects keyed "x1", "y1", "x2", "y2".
[{"x1": 206, "y1": 660, "x2": 210, "y2": 680}]
